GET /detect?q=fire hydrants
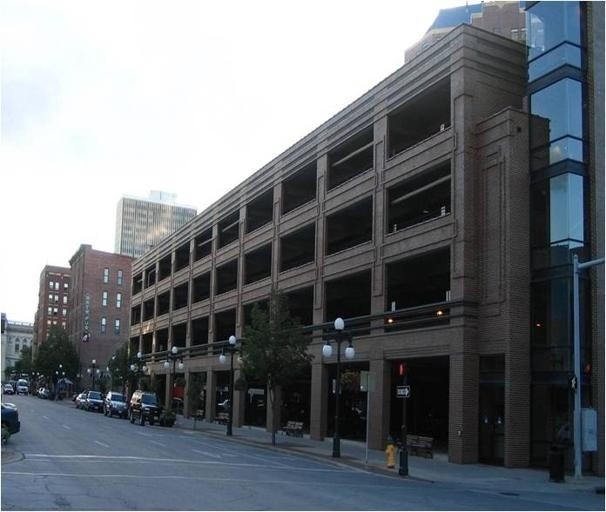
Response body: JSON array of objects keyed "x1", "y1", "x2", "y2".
[{"x1": 384, "y1": 443, "x2": 397, "y2": 468}]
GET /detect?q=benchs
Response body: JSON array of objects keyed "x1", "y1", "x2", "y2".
[
  {"x1": 192, "y1": 409, "x2": 204, "y2": 421},
  {"x1": 214, "y1": 412, "x2": 230, "y2": 425},
  {"x1": 400, "y1": 434, "x2": 435, "y2": 458},
  {"x1": 281, "y1": 421, "x2": 304, "y2": 438}
]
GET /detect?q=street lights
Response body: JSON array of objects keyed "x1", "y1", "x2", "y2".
[
  {"x1": 55, "y1": 362, "x2": 65, "y2": 399},
  {"x1": 129, "y1": 351, "x2": 147, "y2": 394},
  {"x1": 322, "y1": 316, "x2": 355, "y2": 458},
  {"x1": 164, "y1": 345, "x2": 183, "y2": 409},
  {"x1": 86, "y1": 358, "x2": 100, "y2": 391},
  {"x1": 219, "y1": 335, "x2": 245, "y2": 437}
]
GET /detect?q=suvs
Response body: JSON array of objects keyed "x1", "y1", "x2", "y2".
[{"x1": 128, "y1": 390, "x2": 161, "y2": 425}]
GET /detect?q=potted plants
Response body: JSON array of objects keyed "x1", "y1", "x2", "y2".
[{"x1": 164, "y1": 409, "x2": 177, "y2": 427}]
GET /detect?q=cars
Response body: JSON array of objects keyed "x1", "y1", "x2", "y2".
[
  {"x1": 2, "y1": 379, "x2": 29, "y2": 395},
  {"x1": 1, "y1": 402, "x2": 20, "y2": 437},
  {"x1": 74, "y1": 389, "x2": 104, "y2": 412},
  {"x1": 103, "y1": 391, "x2": 127, "y2": 418}
]
